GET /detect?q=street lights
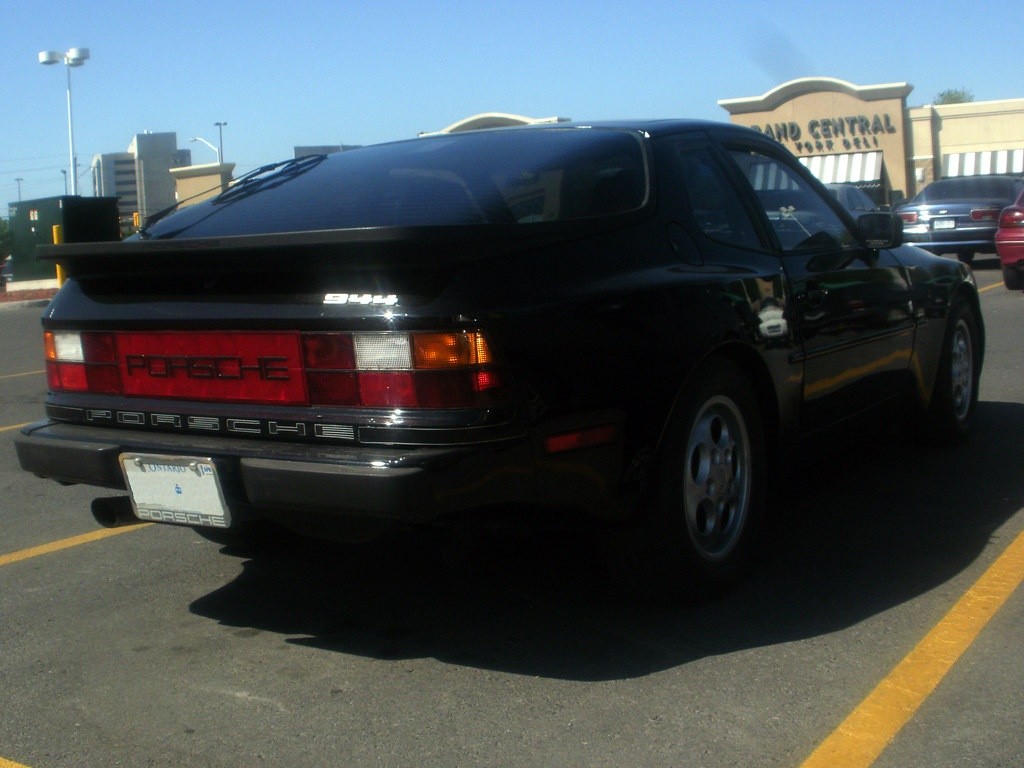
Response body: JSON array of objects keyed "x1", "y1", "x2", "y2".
[
  {"x1": 188, "y1": 137, "x2": 220, "y2": 163},
  {"x1": 15, "y1": 178, "x2": 23, "y2": 202},
  {"x1": 214, "y1": 122, "x2": 227, "y2": 163},
  {"x1": 38, "y1": 47, "x2": 89, "y2": 196},
  {"x1": 60, "y1": 169, "x2": 67, "y2": 195}
]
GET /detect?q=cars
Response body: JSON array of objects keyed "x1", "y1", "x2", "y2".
[
  {"x1": 897, "y1": 175, "x2": 1024, "y2": 263},
  {"x1": 765, "y1": 184, "x2": 878, "y2": 251},
  {"x1": 994, "y1": 188, "x2": 1024, "y2": 290},
  {"x1": 13, "y1": 117, "x2": 986, "y2": 579}
]
